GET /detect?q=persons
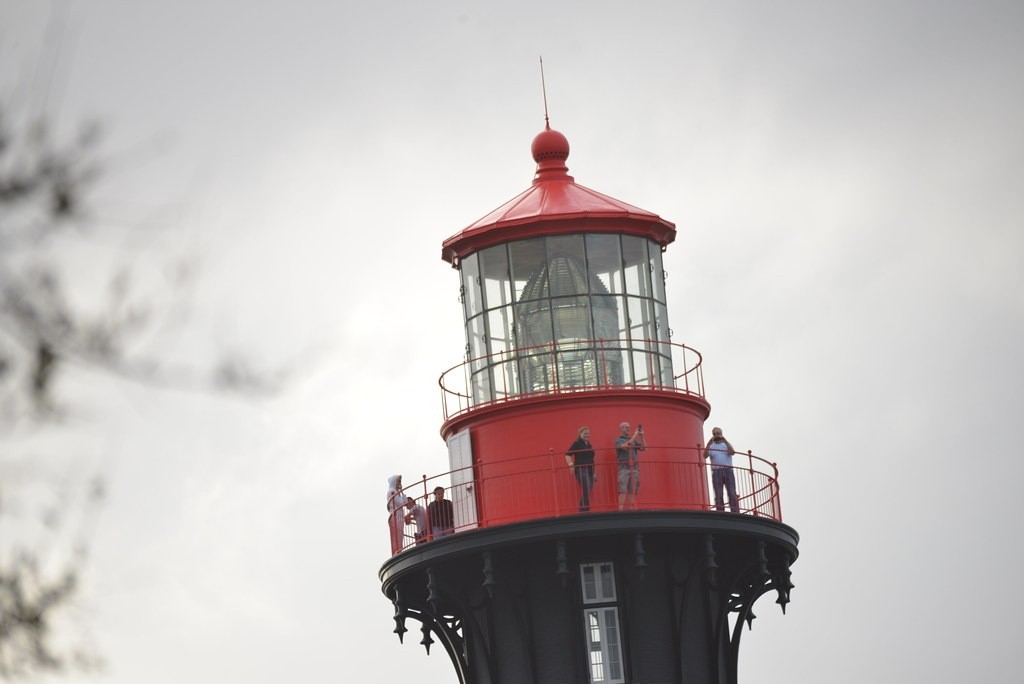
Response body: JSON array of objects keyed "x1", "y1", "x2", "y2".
[
  {"x1": 405, "y1": 497, "x2": 427, "y2": 545},
  {"x1": 427, "y1": 487, "x2": 454, "y2": 540},
  {"x1": 703, "y1": 427, "x2": 739, "y2": 512},
  {"x1": 565, "y1": 426, "x2": 597, "y2": 511},
  {"x1": 615, "y1": 422, "x2": 647, "y2": 510}
]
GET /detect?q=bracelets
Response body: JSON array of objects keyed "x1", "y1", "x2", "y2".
[
  {"x1": 568, "y1": 463, "x2": 573, "y2": 465},
  {"x1": 632, "y1": 436, "x2": 636, "y2": 440}
]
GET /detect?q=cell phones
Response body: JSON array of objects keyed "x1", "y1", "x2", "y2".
[{"x1": 638, "y1": 424, "x2": 642, "y2": 432}]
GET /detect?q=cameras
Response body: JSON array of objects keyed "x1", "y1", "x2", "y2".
[{"x1": 714, "y1": 437, "x2": 721, "y2": 441}]
